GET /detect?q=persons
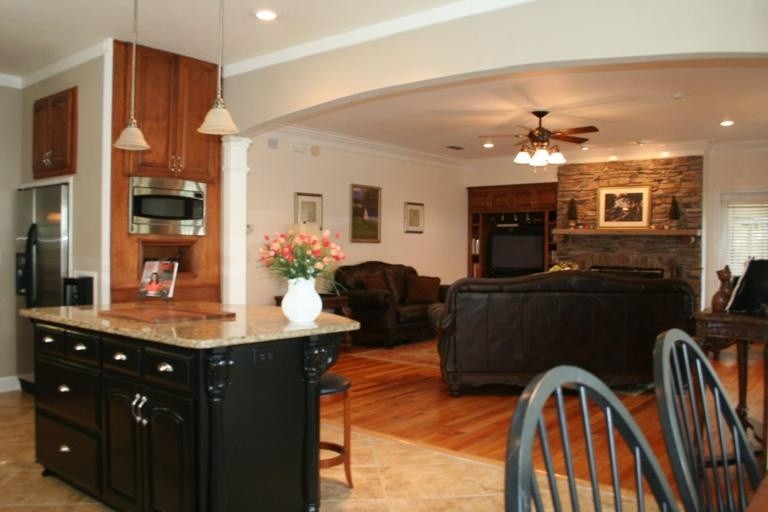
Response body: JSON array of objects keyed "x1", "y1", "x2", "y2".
[{"x1": 138, "y1": 272, "x2": 168, "y2": 297}]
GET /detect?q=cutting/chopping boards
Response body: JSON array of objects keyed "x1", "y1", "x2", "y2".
[{"x1": 96, "y1": 304, "x2": 237, "y2": 324}]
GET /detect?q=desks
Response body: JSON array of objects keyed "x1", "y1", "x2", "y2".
[{"x1": 690, "y1": 311, "x2": 767, "y2": 479}]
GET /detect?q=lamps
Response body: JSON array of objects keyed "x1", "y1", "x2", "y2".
[
  {"x1": 513, "y1": 142, "x2": 567, "y2": 167},
  {"x1": 113, "y1": 0, "x2": 150, "y2": 151},
  {"x1": 197, "y1": 0, "x2": 239, "y2": 135}
]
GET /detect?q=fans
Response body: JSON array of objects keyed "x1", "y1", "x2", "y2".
[{"x1": 477, "y1": 111, "x2": 599, "y2": 147}]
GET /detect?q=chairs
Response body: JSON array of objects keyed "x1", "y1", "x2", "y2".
[
  {"x1": 505, "y1": 365, "x2": 679, "y2": 512},
  {"x1": 652, "y1": 328, "x2": 764, "y2": 512}
]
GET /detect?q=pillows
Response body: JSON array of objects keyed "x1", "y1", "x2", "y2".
[{"x1": 345, "y1": 267, "x2": 440, "y2": 305}]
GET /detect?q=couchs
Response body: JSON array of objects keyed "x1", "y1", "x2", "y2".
[
  {"x1": 332, "y1": 261, "x2": 451, "y2": 349},
  {"x1": 437, "y1": 270, "x2": 697, "y2": 397}
]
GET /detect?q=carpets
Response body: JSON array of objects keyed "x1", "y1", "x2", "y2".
[{"x1": 350, "y1": 339, "x2": 440, "y2": 368}]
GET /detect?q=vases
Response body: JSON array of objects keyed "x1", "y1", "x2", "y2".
[{"x1": 281, "y1": 278, "x2": 323, "y2": 323}]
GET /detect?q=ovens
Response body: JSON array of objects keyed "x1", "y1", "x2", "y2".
[{"x1": 127, "y1": 175, "x2": 208, "y2": 236}]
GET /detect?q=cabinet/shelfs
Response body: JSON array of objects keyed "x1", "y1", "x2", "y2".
[
  {"x1": 110, "y1": 40, "x2": 223, "y2": 305},
  {"x1": 31, "y1": 320, "x2": 339, "y2": 512},
  {"x1": 33, "y1": 85, "x2": 78, "y2": 179}
]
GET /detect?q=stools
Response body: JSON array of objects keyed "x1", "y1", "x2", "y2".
[{"x1": 319, "y1": 372, "x2": 353, "y2": 488}]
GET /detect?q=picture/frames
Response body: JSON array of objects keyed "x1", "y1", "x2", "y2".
[
  {"x1": 350, "y1": 183, "x2": 382, "y2": 243},
  {"x1": 293, "y1": 192, "x2": 323, "y2": 230},
  {"x1": 596, "y1": 183, "x2": 653, "y2": 228},
  {"x1": 404, "y1": 201, "x2": 425, "y2": 233}
]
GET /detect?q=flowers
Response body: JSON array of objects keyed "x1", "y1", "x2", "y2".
[{"x1": 254, "y1": 228, "x2": 349, "y2": 297}]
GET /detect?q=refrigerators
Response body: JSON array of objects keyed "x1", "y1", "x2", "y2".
[{"x1": 13, "y1": 184, "x2": 68, "y2": 396}]
